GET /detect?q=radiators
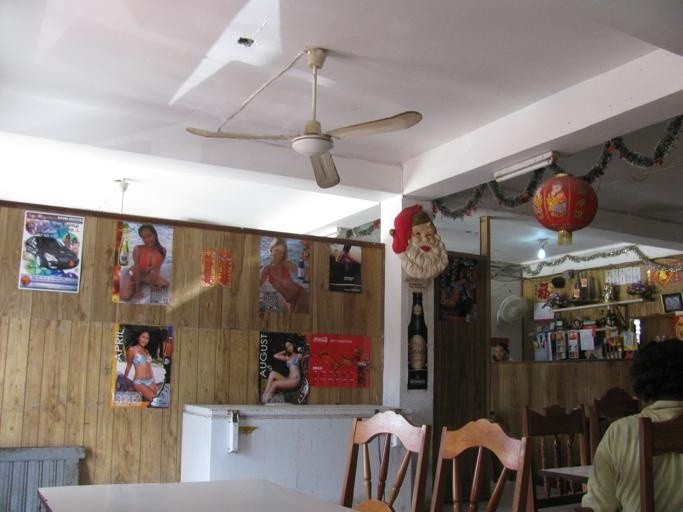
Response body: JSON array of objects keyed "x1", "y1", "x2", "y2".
[{"x1": 0, "y1": 447, "x2": 86, "y2": 512}]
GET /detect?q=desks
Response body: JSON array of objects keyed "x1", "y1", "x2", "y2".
[
  {"x1": 36, "y1": 477, "x2": 353, "y2": 512},
  {"x1": 539, "y1": 462, "x2": 592, "y2": 484}
]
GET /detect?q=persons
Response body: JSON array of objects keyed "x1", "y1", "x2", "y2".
[
  {"x1": 333, "y1": 244, "x2": 357, "y2": 284},
  {"x1": 259, "y1": 238, "x2": 308, "y2": 313},
  {"x1": 491, "y1": 342, "x2": 514, "y2": 362},
  {"x1": 123, "y1": 332, "x2": 158, "y2": 401},
  {"x1": 581, "y1": 338, "x2": 683, "y2": 512},
  {"x1": 120, "y1": 224, "x2": 170, "y2": 301},
  {"x1": 262, "y1": 339, "x2": 307, "y2": 403}
]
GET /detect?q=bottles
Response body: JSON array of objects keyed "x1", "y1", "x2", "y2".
[
  {"x1": 547, "y1": 310, "x2": 623, "y2": 360},
  {"x1": 572, "y1": 280, "x2": 584, "y2": 305},
  {"x1": 408, "y1": 291, "x2": 428, "y2": 390}
]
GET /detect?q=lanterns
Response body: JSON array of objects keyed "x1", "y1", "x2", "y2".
[{"x1": 530, "y1": 172, "x2": 600, "y2": 245}]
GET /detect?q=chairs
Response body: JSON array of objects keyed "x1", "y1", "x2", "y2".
[
  {"x1": 340, "y1": 409, "x2": 432, "y2": 512},
  {"x1": 520, "y1": 404, "x2": 590, "y2": 511},
  {"x1": 639, "y1": 408, "x2": 683, "y2": 512},
  {"x1": 429, "y1": 419, "x2": 528, "y2": 512},
  {"x1": 590, "y1": 388, "x2": 640, "y2": 464}
]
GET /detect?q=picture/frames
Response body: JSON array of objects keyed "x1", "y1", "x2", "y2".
[{"x1": 660, "y1": 290, "x2": 683, "y2": 315}]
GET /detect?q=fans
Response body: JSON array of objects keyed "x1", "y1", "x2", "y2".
[
  {"x1": 496, "y1": 294, "x2": 528, "y2": 329},
  {"x1": 185, "y1": 46, "x2": 423, "y2": 189}
]
